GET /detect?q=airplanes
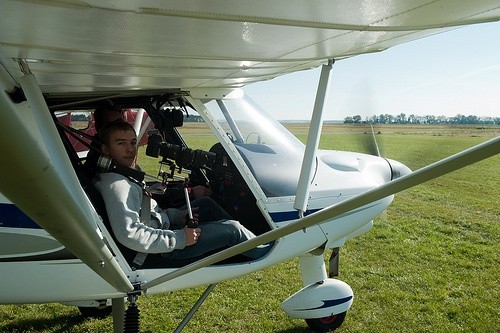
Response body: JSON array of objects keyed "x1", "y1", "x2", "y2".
[
  {"x1": 0, "y1": 1, "x2": 500, "y2": 333},
  {"x1": 55, "y1": 108, "x2": 163, "y2": 153}
]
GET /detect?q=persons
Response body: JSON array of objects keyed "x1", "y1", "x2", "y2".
[
  {"x1": 94, "y1": 120, "x2": 274, "y2": 260},
  {"x1": 82, "y1": 105, "x2": 212, "y2": 240}
]
